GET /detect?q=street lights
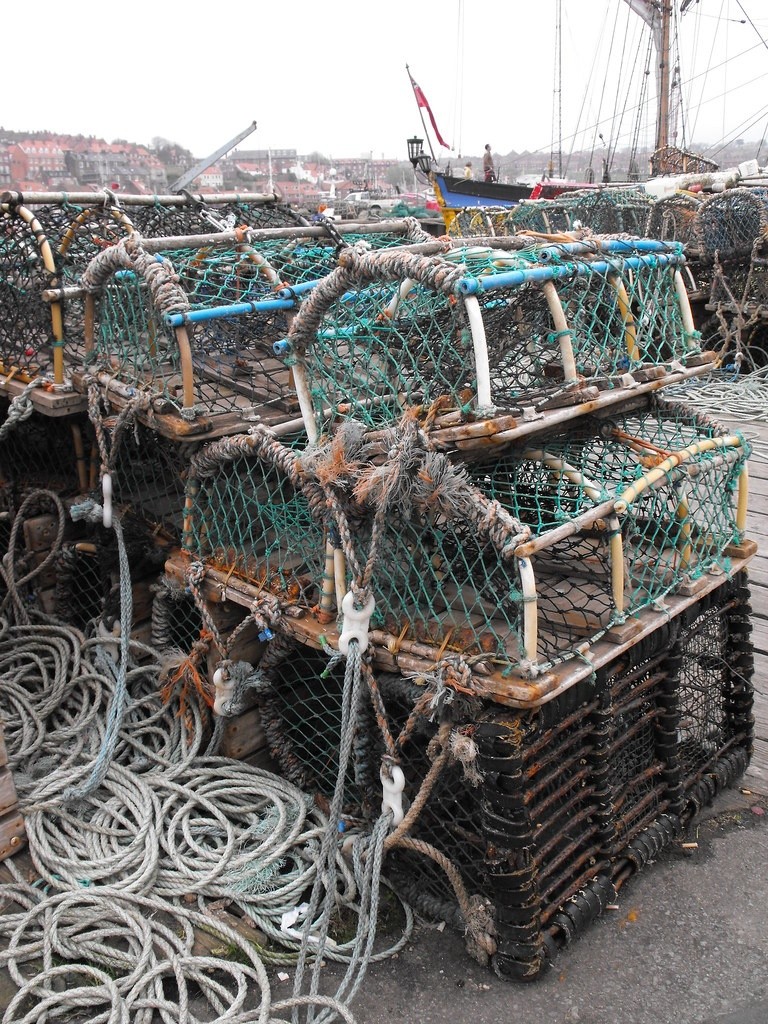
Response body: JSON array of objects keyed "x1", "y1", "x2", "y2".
[{"x1": 407, "y1": 136, "x2": 425, "y2": 214}]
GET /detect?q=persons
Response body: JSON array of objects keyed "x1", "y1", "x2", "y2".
[
  {"x1": 484, "y1": 144, "x2": 496, "y2": 182},
  {"x1": 465, "y1": 162, "x2": 472, "y2": 178}
]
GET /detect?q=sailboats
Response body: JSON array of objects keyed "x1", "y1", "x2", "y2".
[{"x1": 409, "y1": 1, "x2": 768, "y2": 236}]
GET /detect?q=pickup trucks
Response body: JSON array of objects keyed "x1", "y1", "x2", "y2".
[{"x1": 344, "y1": 191, "x2": 403, "y2": 216}]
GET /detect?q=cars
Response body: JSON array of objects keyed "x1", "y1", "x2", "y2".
[{"x1": 389, "y1": 193, "x2": 439, "y2": 213}]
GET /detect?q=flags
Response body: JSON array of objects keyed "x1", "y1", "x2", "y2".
[{"x1": 412, "y1": 79, "x2": 451, "y2": 150}]
